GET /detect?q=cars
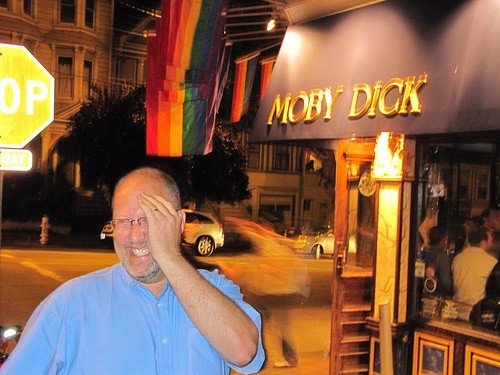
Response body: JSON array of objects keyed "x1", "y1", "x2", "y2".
[
  {"x1": 295, "y1": 226, "x2": 374, "y2": 260},
  {"x1": 99, "y1": 207, "x2": 225, "y2": 257}
]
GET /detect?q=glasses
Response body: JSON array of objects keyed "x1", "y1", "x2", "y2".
[{"x1": 110, "y1": 218, "x2": 150, "y2": 231}]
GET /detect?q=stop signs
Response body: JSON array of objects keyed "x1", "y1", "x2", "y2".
[{"x1": 0, "y1": 42, "x2": 55, "y2": 148}]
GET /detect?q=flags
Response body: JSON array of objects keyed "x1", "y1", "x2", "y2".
[
  {"x1": 230, "y1": 52, "x2": 260, "y2": 124},
  {"x1": 260, "y1": 60, "x2": 277, "y2": 102},
  {"x1": 145, "y1": 0, "x2": 232, "y2": 157}
]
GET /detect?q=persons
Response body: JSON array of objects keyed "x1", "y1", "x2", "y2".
[
  {"x1": 420, "y1": 203, "x2": 500, "y2": 332},
  {"x1": 0, "y1": 166, "x2": 265, "y2": 375}
]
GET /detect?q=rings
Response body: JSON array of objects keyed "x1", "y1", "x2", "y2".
[{"x1": 153, "y1": 208, "x2": 159, "y2": 215}]
